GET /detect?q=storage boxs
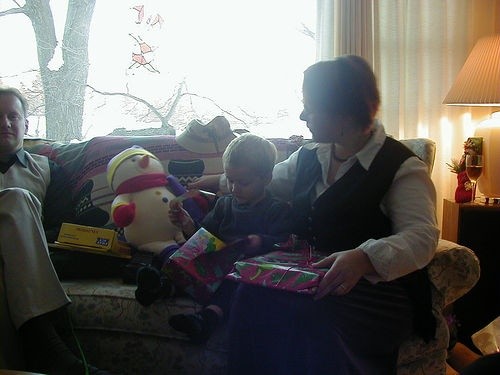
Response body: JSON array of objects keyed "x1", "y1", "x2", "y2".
[
  {"x1": 54, "y1": 223, "x2": 133, "y2": 256},
  {"x1": 224, "y1": 247, "x2": 333, "y2": 294},
  {"x1": 170, "y1": 227, "x2": 228, "y2": 294}
]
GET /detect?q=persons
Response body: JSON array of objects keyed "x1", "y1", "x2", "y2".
[
  {"x1": 0, "y1": 87, "x2": 113, "y2": 375},
  {"x1": 135, "y1": 134, "x2": 295, "y2": 345},
  {"x1": 187, "y1": 54, "x2": 440, "y2": 375}
]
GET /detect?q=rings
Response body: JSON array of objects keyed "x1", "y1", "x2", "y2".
[{"x1": 340, "y1": 284, "x2": 347, "y2": 292}]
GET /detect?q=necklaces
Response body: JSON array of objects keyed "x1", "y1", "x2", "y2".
[{"x1": 332, "y1": 144, "x2": 351, "y2": 162}]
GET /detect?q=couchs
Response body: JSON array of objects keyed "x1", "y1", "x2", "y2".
[{"x1": 21, "y1": 138, "x2": 481, "y2": 375}]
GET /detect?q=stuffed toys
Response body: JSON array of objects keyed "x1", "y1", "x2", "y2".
[{"x1": 107, "y1": 144, "x2": 187, "y2": 259}]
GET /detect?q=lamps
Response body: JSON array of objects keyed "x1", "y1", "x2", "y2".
[{"x1": 442, "y1": 34, "x2": 500, "y2": 204}]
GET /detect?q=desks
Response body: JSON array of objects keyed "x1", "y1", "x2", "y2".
[{"x1": 442, "y1": 198, "x2": 500, "y2": 244}]
